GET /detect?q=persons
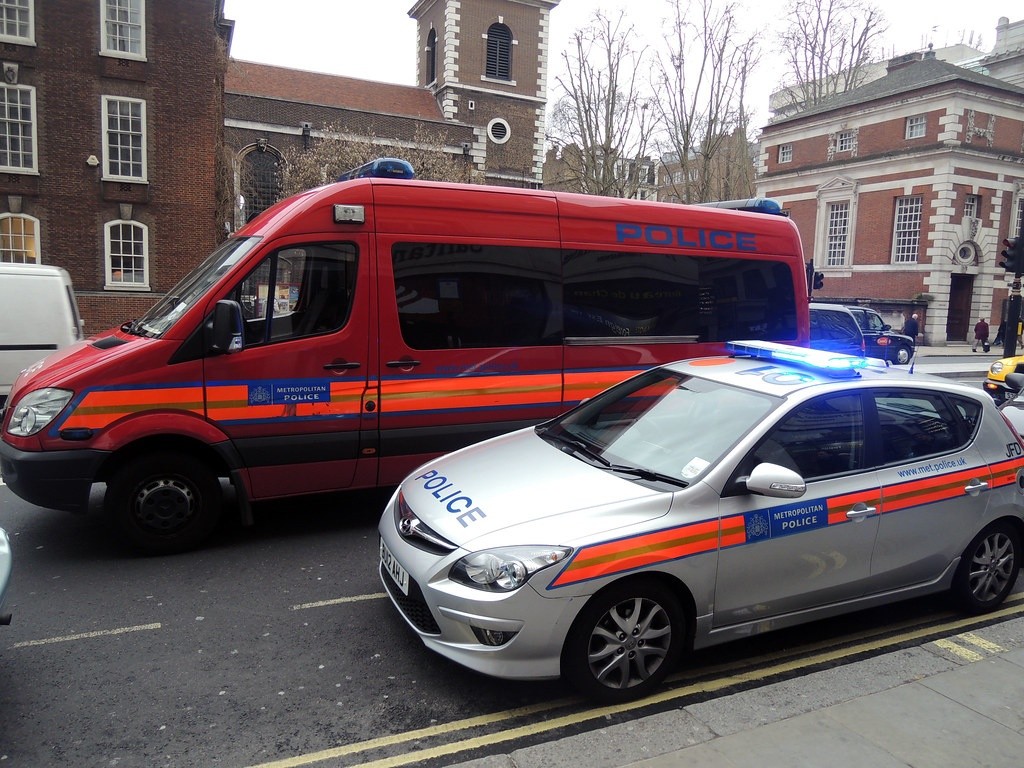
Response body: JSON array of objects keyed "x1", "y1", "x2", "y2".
[
  {"x1": 993, "y1": 318, "x2": 1007, "y2": 348},
  {"x1": 972, "y1": 317, "x2": 989, "y2": 352},
  {"x1": 1018, "y1": 316, "x2": 1024, "y2": 349},
  {"x1": 904, "y1": 314, "x2": 919, "y2": 352}
]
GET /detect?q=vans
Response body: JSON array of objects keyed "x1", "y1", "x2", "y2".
[
  {"x1": 0, "y1": 264, "x2": 84, "y2": 400},
  {"x1": 0, "y1": 158, "x2": 822, "y2": 560}
]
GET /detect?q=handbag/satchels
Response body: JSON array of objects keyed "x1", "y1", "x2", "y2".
[{"x1": 983, "y1": 339, "x2": 990, "y2": 352}]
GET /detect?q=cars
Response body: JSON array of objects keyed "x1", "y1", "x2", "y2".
[
  {"x1": 847, "y1": 306, "x2": 915, "y2": 365},
  {"x1": 809, "y1": 302, "x2": 866, "y2": 359},
  {"x1": 372, "y1": 339, "x2": 1020, "y2": 708},
  {"x1": 998, "y1": 388, "x2": 1024, "y2": 439},
  {"x1": 982, "y1": 352, "x2": 1024, "y2": 402}
]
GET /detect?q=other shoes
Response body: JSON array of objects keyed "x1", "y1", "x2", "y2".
[
  {"x1": 1021, "y1": 345, "x2": 1024, "y2": 349},
  {"x1": 973, "y1": 349, "x2": 977, "y2": 352}
]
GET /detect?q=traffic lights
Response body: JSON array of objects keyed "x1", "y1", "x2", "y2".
[{"x1": 998, "y1": 236, "x2": 1024, "y2": 274}]
